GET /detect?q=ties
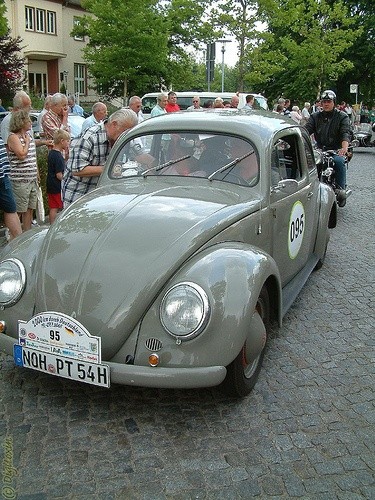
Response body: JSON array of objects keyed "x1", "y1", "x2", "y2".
[{"x1": 71, "y1": 107, "x2": 73, "y2": 113}]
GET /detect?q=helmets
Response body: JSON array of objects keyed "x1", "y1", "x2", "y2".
[{"x1": 319, "y1": 90, "x2": 336, "y2": 102}]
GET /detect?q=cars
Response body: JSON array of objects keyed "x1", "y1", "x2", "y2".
[{"x1": 0, "y1": 108, "x2": 340, "y2": 398}]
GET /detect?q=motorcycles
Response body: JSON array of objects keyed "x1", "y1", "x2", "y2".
[
  {"x1": 280, "y1": 139, "x2": 360, "y2": 209},
  {"x1": 351, "y1": 114, "x2": 375, "y2": 147}
]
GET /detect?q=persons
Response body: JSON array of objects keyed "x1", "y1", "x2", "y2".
[
  {"x1": 0, "y1": 91, "x2": 375, "y2": 240},
  {"x1": 303, "y1": 90, "x2": 351, "y2": 207}
]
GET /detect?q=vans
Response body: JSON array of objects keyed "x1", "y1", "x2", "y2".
[{"x1": 133, "y1": 91, "x2": 269, "y2": 143}]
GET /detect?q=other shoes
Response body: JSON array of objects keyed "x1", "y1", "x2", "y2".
[{"x1": 335, "y1": 189, "x2": 347, "y2": 208}]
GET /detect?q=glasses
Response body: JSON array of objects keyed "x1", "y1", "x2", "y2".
[{"x1": 193, "y1": 101, "x2": 198, "y2": 103}]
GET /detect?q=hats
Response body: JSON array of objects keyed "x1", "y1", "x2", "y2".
[{"x1": 304, "y1": 102, "x2": 310, "y2": 107}]
